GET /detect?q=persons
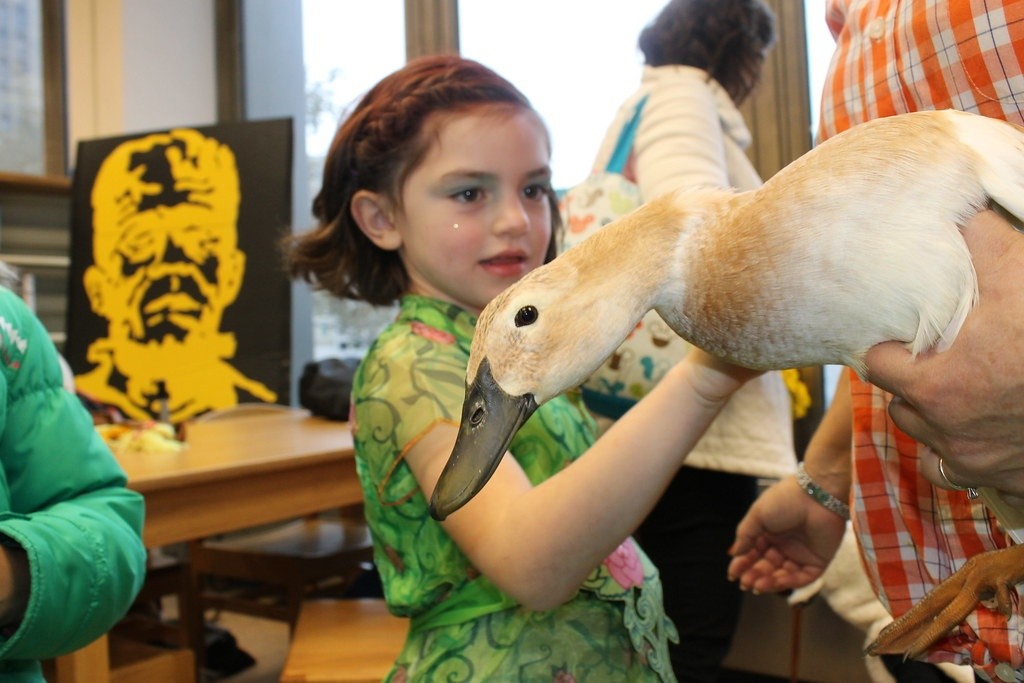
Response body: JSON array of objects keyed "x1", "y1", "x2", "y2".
[
  {"x1": 726, "y1": 0, "x2": 1024, "y2": 683},
  {"x1": 559, "y1": 0, "x2": 797, "y2": 683},
  {"x1": 283, "y1": 56, "x2": 771, "y2": 683},
  {"x1": 0, "y1": 284, "x2": 147, "y2": 683}
]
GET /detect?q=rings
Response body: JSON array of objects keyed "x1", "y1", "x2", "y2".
[{"x1": 938, "y1": 457, "x2": 981, "y2": 500}]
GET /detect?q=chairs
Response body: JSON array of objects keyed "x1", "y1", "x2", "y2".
[{"x1": 111, "y1": 404, "x2": 411, "y2": 683}]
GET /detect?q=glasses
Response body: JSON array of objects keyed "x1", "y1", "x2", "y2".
[{"x1": 746, "y1": 47, "x2": 768, "y2": 63}]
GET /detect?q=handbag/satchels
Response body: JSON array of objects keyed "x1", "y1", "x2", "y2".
[{"x1": 555, "y1": 91, "x2": 677, "y2": 416}]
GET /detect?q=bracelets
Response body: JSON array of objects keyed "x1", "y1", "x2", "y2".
[{"x1": 794, "y1": 461, "x2": 851, "y2": 521}]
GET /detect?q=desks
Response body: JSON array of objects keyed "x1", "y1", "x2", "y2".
[{"x1": 42, "y1": 402, "x2": 365, "y2": 683}]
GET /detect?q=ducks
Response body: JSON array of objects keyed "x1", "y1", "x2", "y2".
[{"x1": 427, "y1": 106, "x2": 1024, "y2": 665}]
